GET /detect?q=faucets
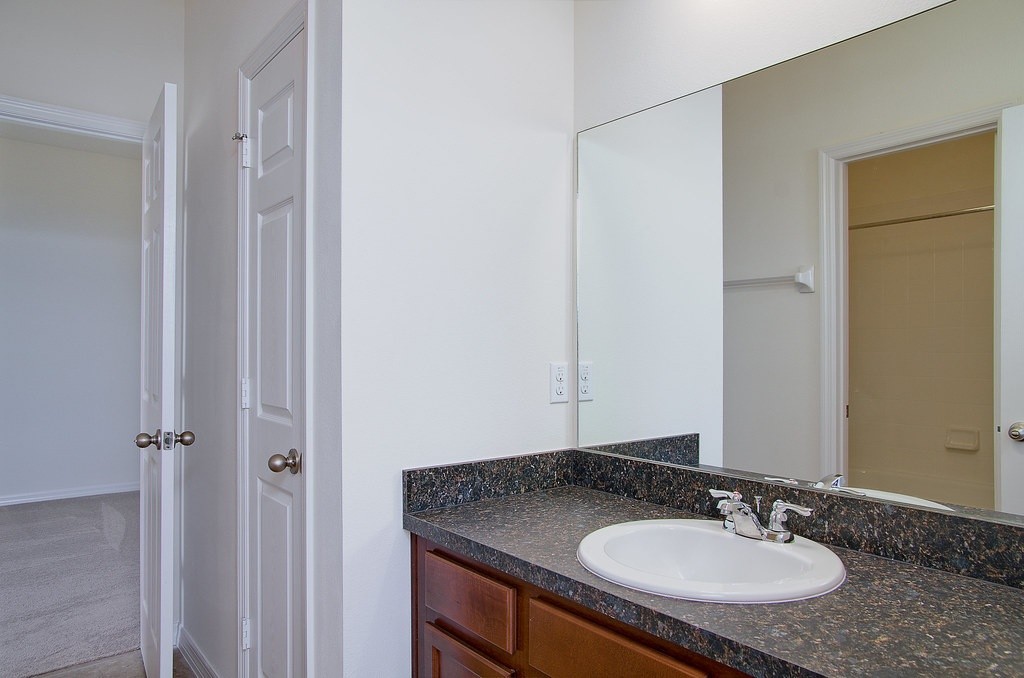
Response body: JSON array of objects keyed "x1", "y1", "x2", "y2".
[
  {"x1": 764, "y1": 473, "x2": 866, "y2": 499},
  {"x1": 708, "y1": 488, "x2": 817, "y2": 544}
]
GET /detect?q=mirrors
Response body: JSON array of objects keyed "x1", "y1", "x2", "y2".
[{"x1": 575, "y1": 0, "x2": 1024, "y2": 519}]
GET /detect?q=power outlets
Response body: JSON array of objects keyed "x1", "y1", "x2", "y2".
[
  {"x1": 550, "y1": 363, "x2": 568, "y2": 403},
  {"x1": 577, "y1": 364, "x2": 594, "y2": 401}
]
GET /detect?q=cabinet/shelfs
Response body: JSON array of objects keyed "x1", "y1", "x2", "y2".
[{"x1": 408, "y1": 533, "x2": 752, "y2": 678}]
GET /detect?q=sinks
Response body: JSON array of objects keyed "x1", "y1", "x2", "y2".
[
  {"x1": 575, "y1": 518, "x2": 847, "y2": 605},
  {"x1": 839, "y1": 487, "x2": 957, "y2": 512}
]
